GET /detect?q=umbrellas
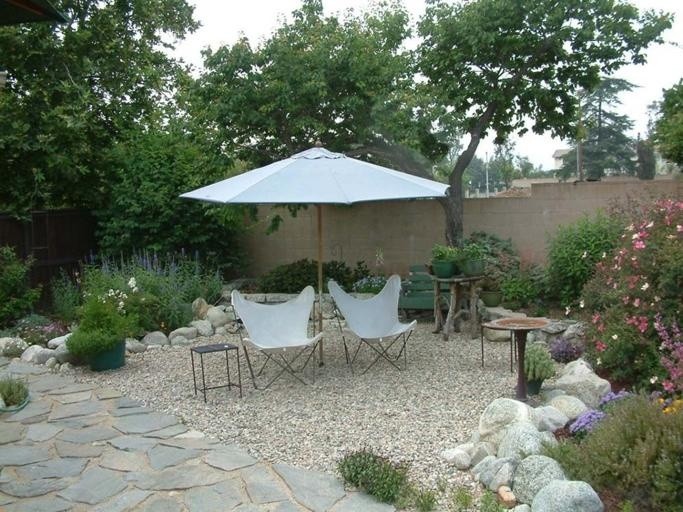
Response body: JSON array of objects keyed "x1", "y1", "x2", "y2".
[{"x1": 179, "y1": 138, "x2": 451, "y2": 367}]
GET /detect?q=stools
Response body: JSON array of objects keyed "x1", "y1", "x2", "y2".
[{"x1": 188, "y1": 341, "x2": 243, "y2": 401}]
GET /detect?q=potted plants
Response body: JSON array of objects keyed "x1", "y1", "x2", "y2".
[
  {"x1": 462, "y1": 247, "x2": 486, "y2": 275},
  {"x1": 65, "y1": 298, "x2": 139, "y2": 371},
  {"x1": 431, "y1": 245, "x2": 461, "y2": 278},
  {"x1": 522, "y1": 347, "x2": 556, "y2": 395}
]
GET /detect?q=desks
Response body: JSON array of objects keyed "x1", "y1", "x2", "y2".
[
  {"x1": 485, "y1": 318, "x2": 552, "y2": 402},
  {"x1": 430, "y1": 276, "x2": 482, "y2": 342}
]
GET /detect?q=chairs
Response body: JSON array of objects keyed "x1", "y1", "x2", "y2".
[
  {"x1": 324, "y1": 273, "x2": 417, "y2": 374},
  {"x1": 475, "y1": 305, "x2": 514, "y2": 370},
  {"x1": 228, "y1": 284, "x2": 328, "y2": 391}
]
